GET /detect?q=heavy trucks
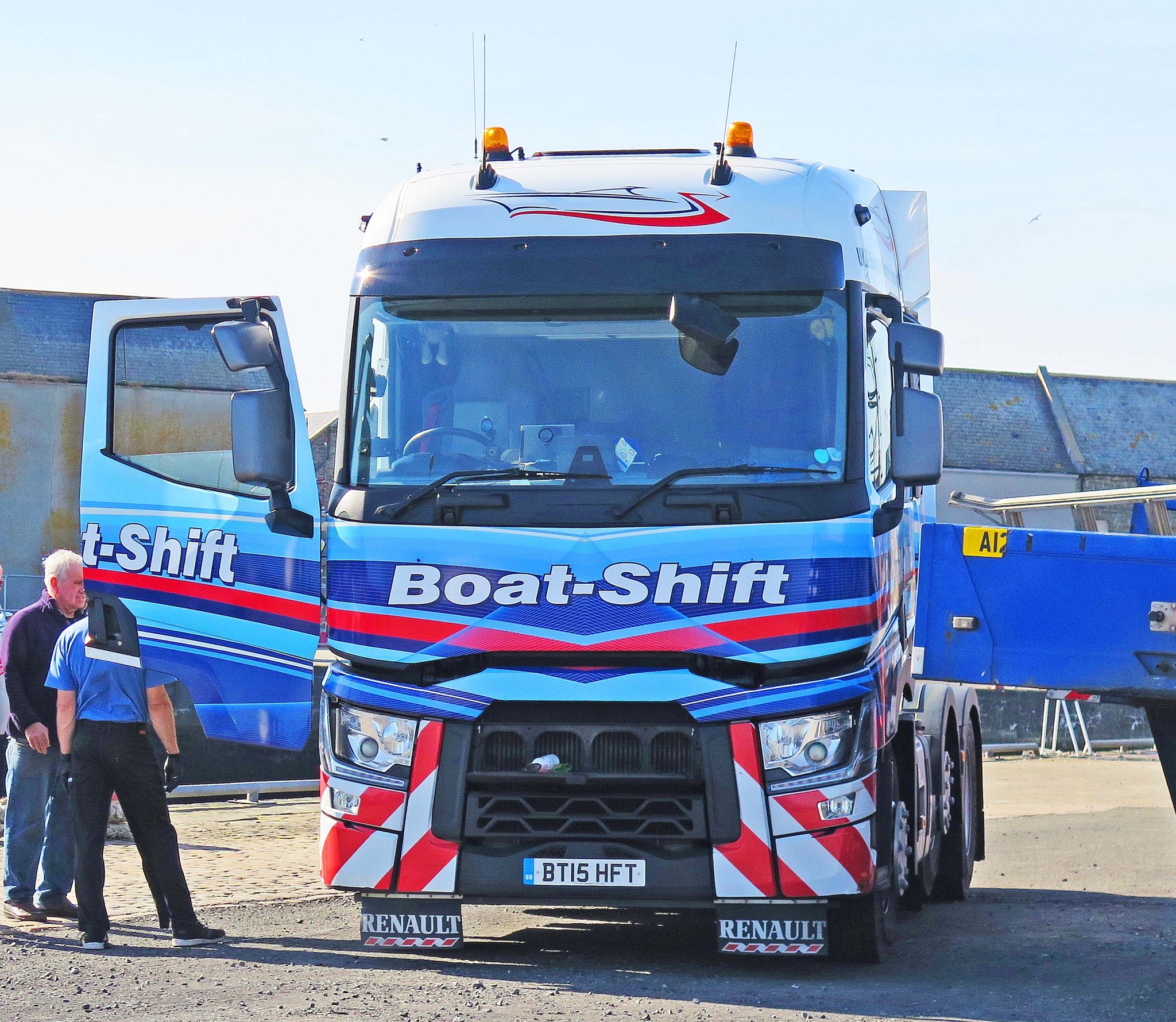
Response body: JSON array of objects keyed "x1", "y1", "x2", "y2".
[{"x1": 79, "y1": 121, "x2": 1176, "y2": 965}]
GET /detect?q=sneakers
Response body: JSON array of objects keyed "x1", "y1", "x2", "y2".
[
  {"x1": 171, "y1": 923, "x2": 225, "y2": 946},
  {"x1": 77, "y1": 929, "x2": 107, "y2": 949}
]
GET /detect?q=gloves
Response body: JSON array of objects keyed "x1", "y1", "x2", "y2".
[
  {"x1": 60, "y1": 752, "x2": 71, "y2": 793},
  {"x1": 163, "y1": 750, "x2": 182, "y2": 794}
]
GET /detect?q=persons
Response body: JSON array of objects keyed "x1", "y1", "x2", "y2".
[{"x1": 0, "y1": 549, "x2": 225, "y2": 949}]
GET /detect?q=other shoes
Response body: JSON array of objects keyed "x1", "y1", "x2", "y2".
[
  {"x1": 34, "y1": 896, "x2": 78, "y2": 920},
  {"x1": 3, "y1": 900, "x2": 48, "y2": 922}
]
